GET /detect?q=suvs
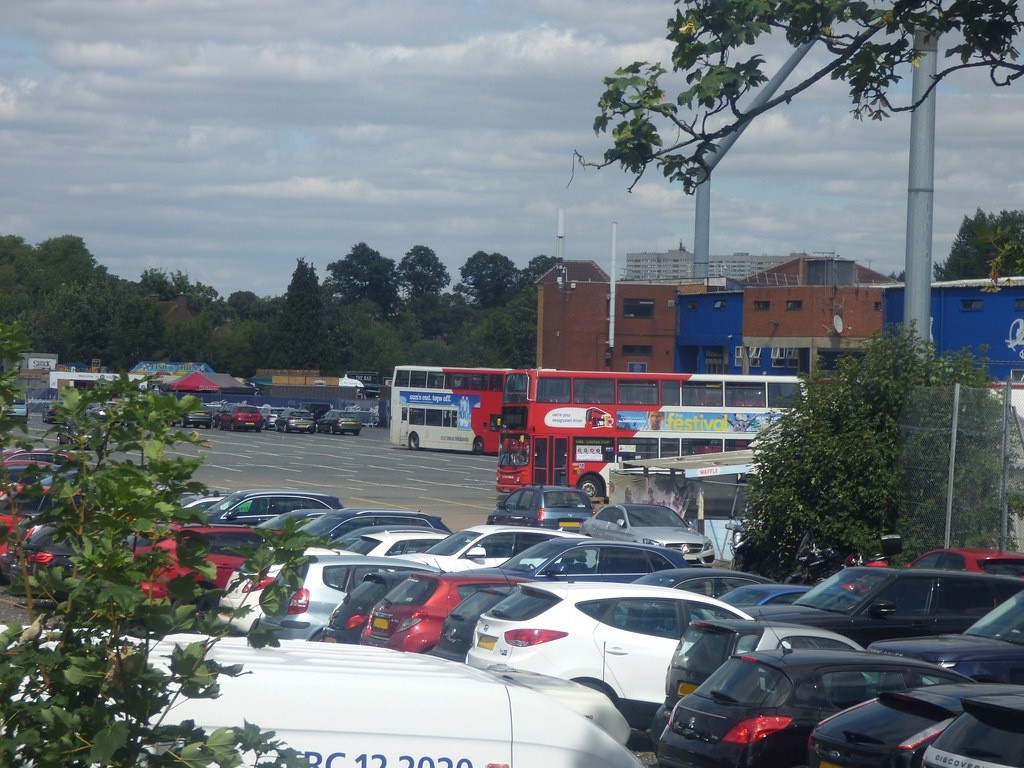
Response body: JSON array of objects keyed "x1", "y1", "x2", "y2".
[
  {"x1": 866, "y1": 589, "x2": 1024, "y2": 687},
  {"x1": 0, "y1": 401, "x2": 1024, "y2": 767},
  {"x1": 714, "y1": 565, "x2": 1024, "y2": 648},
  {"x1": 486, "y1": 481, "x2": 594, "y2": 541}
]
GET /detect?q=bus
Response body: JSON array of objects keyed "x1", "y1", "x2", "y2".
[
  {"x1": 390, "y1": 364, "x2": 556, "y2": 456},
  {"x1": 495, "y1": 367, "x2": 812, "y2": 494}
]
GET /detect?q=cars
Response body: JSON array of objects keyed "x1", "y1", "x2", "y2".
[
  {"x1": 315, "y1": 409, "x2": 362, "y2": 436},
  {"x1": 260, "y1": 407, "x2": 286, "y2": 431},
  {"x1": 168, "y1": 404, "x2": 213, "y2": 429},
  {"x1": 578, "y1": 502, "x2": 716, "y2": 568},
  {"x1": 891, "y1": 548, "x2": 1024, "y2": 585},
  {"x1": 275, "y1": 408, "x2": 317, "y2": 434},
  {"x1": 218, "y1": 404, "x2": 264, "y2": 432}
]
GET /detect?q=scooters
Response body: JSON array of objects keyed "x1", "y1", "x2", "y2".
[
  {"x1": 724, "y1": 512, "x2": 763, "y2": 572},
  {"x1": 783, "y1": 529, "x2": 903, "y2": 586}
]
